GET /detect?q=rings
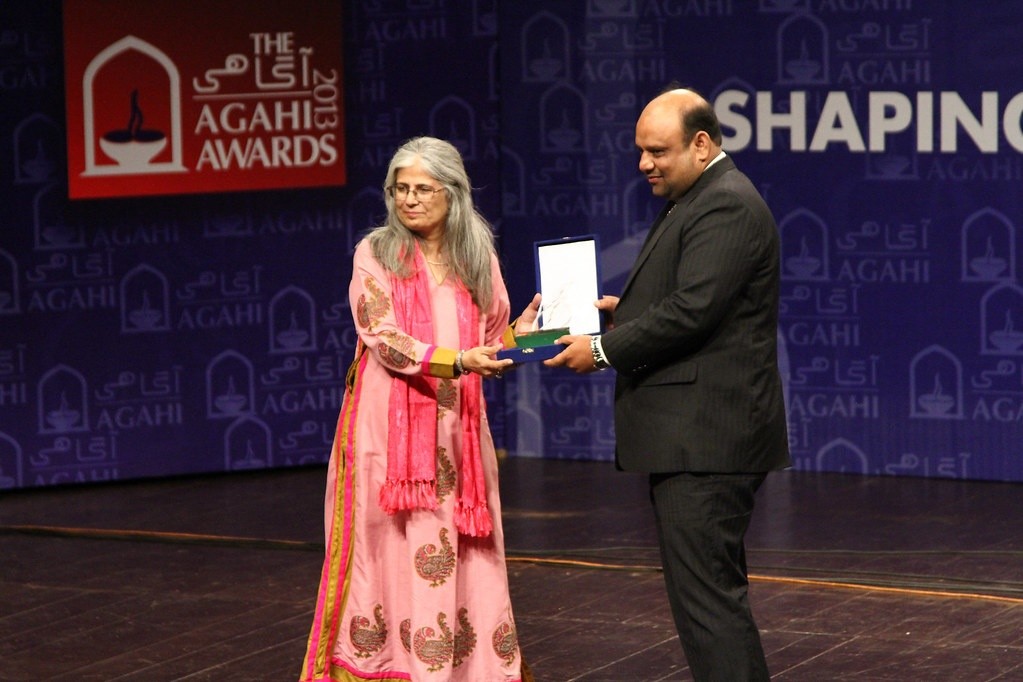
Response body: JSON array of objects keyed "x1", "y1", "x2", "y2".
[{"x1": 495, "y1": 371, "x2": 502, "y2": 379}]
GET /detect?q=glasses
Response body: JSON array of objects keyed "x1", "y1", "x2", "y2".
[{"x1": 386, "y1": 185, "x2": 446, "y2": 201}]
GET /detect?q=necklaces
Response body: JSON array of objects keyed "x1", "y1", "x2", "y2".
[{"x1": 428, "y1": 261, "x2": 449, "y2": 265}]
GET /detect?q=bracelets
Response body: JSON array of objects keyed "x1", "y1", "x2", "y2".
[{"x1": 456, "y1": 350, "x2": 471, "y2": 375}]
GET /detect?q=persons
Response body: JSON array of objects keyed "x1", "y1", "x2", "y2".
[
  {"x1": 299, "y1": 136, "x2": 543, "y2": 682},
  {"x1": 543, "y1": 88, "x2": 791, "y2": 682}
]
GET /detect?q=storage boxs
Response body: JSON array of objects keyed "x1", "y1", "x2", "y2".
[{"x1": 495, "y1": 231, "x2": 605, "y2": 362}]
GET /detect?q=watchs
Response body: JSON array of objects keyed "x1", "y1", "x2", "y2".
[{"x1": 591, "y1": 335, "x2": 610, "y2": 371}]
